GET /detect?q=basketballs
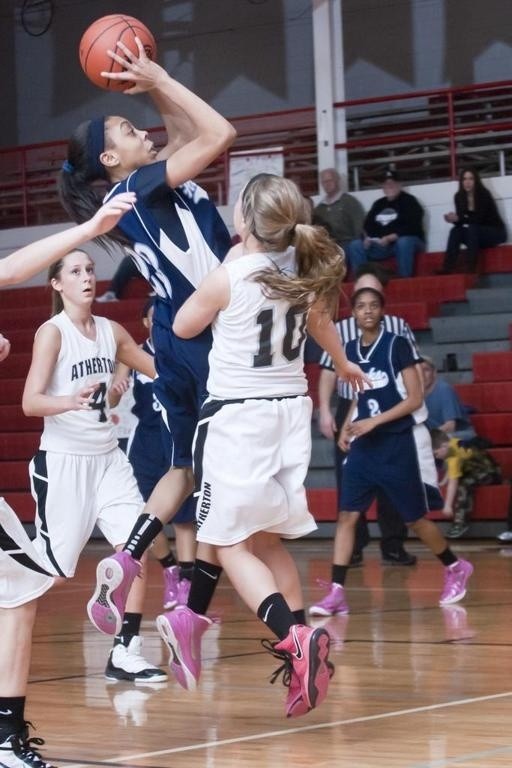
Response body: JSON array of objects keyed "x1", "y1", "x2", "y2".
[{"x1": 79, "y1": 15, "x2": 159, "y2": 93}]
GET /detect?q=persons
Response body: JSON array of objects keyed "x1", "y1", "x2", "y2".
[
  {"x1": 311, "y1": 169, "x2": 427, "y2": 278},
  {"x1": 433, "y1": 168, "x2": 507, "y2": 274},
  {"x1": 308, "y1": 273, "x2": 494, "y2": 615},
  {"x1": 0, "y1": 37, "x2": 347, "y2": 767}
]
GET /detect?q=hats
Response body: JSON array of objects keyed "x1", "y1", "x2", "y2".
[{"x1": 375, "y1": 172, "x2": 400, "y2": 184}]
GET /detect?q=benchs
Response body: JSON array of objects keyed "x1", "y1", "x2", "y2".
[{"x1": 0, "y1": 245, "x2": 512, "y2": 539}]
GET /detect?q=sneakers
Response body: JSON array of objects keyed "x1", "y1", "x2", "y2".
[
  {"x1": 1, "y1": 724, "x2": 55, "y2": 768},
  {"x1": 275, "y1": 625, "x2": 333, "y2": 717},
  {"x1": 498, "y1": 531, "x2": 511, "y2": 542},
  {"x1": 439, "y1": 560, "x2": 474, "y2": 604},
  {"x1": 445, "y1": 520, "x2": 468, "y2": 538},
  {"x1": 162, "y1": 569, "x2": 190, "y2": 611},
  {"x1": 349, "y1": 550, "x2": 362, "y2": 566},
  {"x1": 154, "y1": 610, "x2": 210, "y2": 690},
  {"x1": 381, "y1": 551, "x2": 416, "y2": 567},
  {"x1": 103, "y1": 637, "x2": 169, "y2": 683},
  {"x1": 308, "y1": 585, "x2": 351, "y2": 616},
  {"x1": 86, "y1": 553, "x2": 138, "y2": 636}
]
